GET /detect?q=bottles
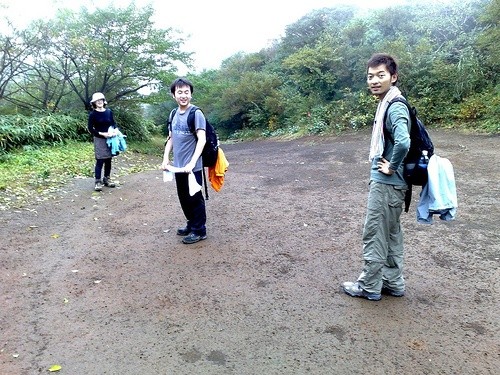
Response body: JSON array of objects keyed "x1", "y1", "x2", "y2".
[{"x1": 418, "y1": 150, "x2": 430, "y2": 169}]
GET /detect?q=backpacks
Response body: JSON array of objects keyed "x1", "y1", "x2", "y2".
[
  {"x1": 169, "y1": 106, "x2": 218, "y2": 167},
  {"x1": 383, "y1": 98, "x2": 433, "y2": 186}
]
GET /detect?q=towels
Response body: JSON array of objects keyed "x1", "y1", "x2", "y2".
[{"x1": 162, "y1": 164, "x2": 202, "y2": 197}]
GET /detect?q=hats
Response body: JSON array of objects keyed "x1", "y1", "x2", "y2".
[{"x1": 90, "y1": 92, "x2": 108, "y2": 105}]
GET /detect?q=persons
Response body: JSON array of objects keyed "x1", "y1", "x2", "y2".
[
  {"x1": 343, "y1": 52, "x2": 413, "y2": 300},
  {"x1": 88, "y1": 92, "x2": 118, "y2": 191},
  {"x1": 160, "y1": 79, "x2": 208, "y2": 244}
]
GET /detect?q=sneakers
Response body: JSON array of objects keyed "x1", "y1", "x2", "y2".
[
  {"x1": 342, "y1": 282, "x2": 381, "y2": 301},
  {"x1": 182, "y1": 232, "x2": 207, "y2": 244},
  {"x1": 177, "y1": 227, "x2": 190, "y2": 235},
  {"x1": 104, "y1": 179, "x2": 115, "y2": 188},
  {"x1": 382, "y1": 287, "x2": 404, "y2": 296},
  {"x1": 94, "y1": 182, "x2": 102, "y2": 190}
]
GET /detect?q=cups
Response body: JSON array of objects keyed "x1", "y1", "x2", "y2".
[{"x1": 407, "y1": 163, "x2": 416, "y2": 176}]
